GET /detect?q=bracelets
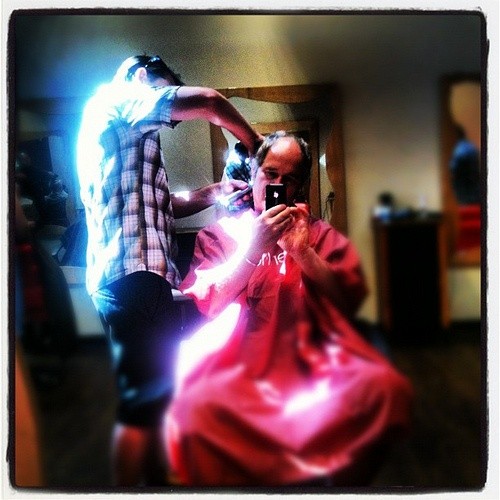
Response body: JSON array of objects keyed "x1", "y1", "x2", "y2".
[{"x1": 241, "y1": 254, "x2": 263, "y2": 266}]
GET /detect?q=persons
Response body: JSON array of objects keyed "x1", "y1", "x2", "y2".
[
  {"x1": 162, "y1": 130, "x2": 412, "y2": 487},
  {"x1": 75, "y1": 53, "x2": 266, "y2": 486}
]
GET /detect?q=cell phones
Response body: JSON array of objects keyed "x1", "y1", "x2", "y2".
[{"x1": 266, "y1": 183, "x2": 286, "y2": 212}]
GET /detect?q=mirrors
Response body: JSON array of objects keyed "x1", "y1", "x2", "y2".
[
  {"x1": 209, "y1": 80, "x2": 348, "y2": 237},
  {"x1": 15, "y1": 131, "x2": 89, "y2": 284},
  {"x1": 435, "y1": 71, "x2": 480, "y2": 268}
]
